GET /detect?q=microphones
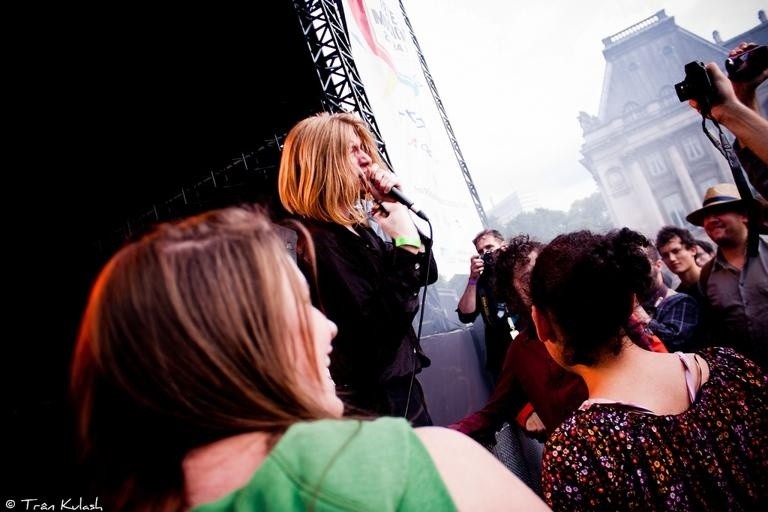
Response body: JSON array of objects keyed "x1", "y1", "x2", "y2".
[{"x1": 386, "y1": 186, "x2": 429, "y2": 222}]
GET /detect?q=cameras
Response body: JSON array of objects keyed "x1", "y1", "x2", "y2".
[
  {"x1": 480, "y1": 251, "x2": 496, "y2": 267},
  {"x1": 725, "y1": 44, "x2": 768, "y2": 82},
  {"x1": 674, "y1": 60, "x2": 717, "y2": 106}
]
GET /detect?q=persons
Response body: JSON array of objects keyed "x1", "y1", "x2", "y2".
[{"x1": 65, "y1": 42, "x2": 767, "y2": 510}]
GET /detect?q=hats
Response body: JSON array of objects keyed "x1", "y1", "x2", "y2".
[{"x1": 686, "y1": 187, "x2": 759, "y2": 227}]
